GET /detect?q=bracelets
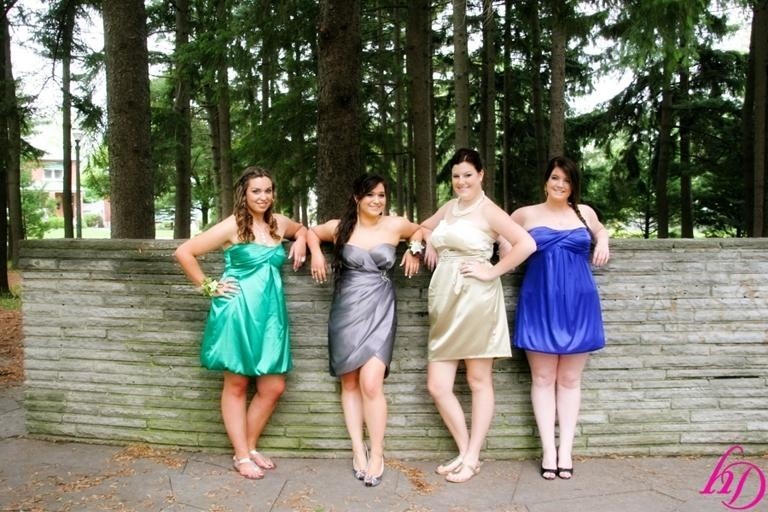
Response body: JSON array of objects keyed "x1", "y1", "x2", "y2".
[
  {"x1": 194, "y1": 276, "x2": 218, "y2": 299},
  {"x1": 408, "y1": 240, "x2": 426, "y2": 255}
]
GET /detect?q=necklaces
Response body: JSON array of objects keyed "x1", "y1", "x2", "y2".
[
  {"x1": 453, "y1": 191, "x2": 484, "y2": 217},
  {"x1": 255, "y1": 221, "x2": 268, "y2": 244}
]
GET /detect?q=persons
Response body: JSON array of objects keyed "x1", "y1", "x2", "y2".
[
  {"x1": 420, "y1": 148, "x2": 537, "y2": 484},
  {"x1": 502, "y1": 157, "x2": 609, "y2": 479},
  {"x1": 307, "y1": 173, "x2": 424, "y2": 487},
  {"x1": 175, "y1": 165, "x2": 308, "y2": 480}
]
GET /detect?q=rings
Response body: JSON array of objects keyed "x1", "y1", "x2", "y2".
[{"x1": 301, "y1": 257, "x2": 305, "y2": 262}]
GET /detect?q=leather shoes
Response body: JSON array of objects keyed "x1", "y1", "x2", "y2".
[{"x1": 352, "y1": 442, "x2": 385, "y2": 487}]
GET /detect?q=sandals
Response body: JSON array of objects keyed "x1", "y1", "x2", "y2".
[
  {"x1": 233, "y1": 449, "x2": 276, "y2": 480},
  {"x1": 541, "y1": 460, "x2": 573, "y2": 479},
  {"x1": 436, "y1": 451, "x2": 480, "y2": 483}
]
